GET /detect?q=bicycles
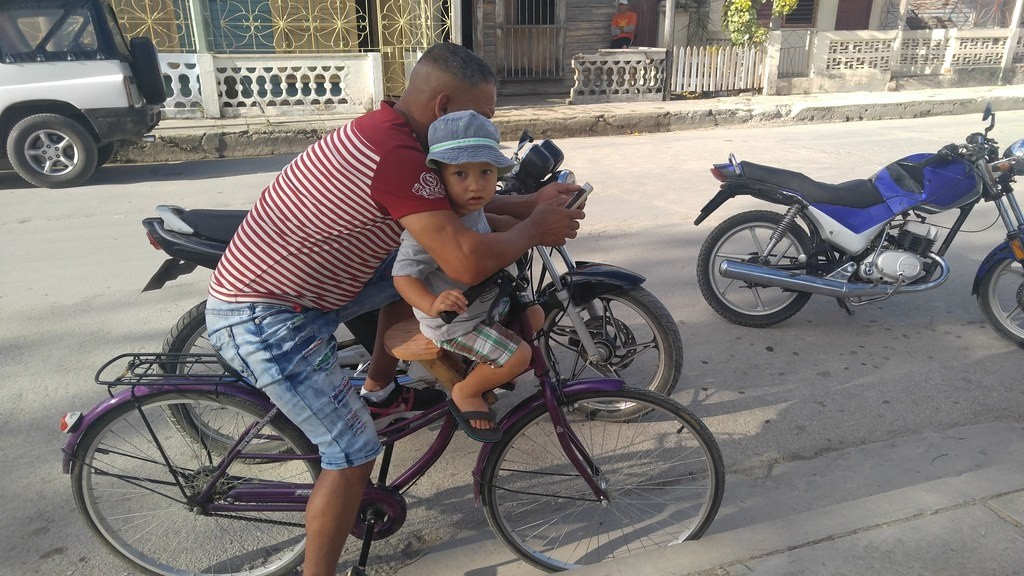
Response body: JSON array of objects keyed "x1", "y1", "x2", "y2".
[{"x1": 61, "y1": 250, "x2": 731, "y2": 576}]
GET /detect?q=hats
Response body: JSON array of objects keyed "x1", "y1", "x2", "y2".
[
  {"x1": 425, "y1": 110, "x2": 516, "y2": 177},
  {"x1": 618, "y1": 0, "x2": 629, "y2": 6}
]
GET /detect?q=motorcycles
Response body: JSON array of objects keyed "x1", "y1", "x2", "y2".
[
  {"x1": 693, "y1": 102, "x2": 1023, "y2": 348},
  {"x1": 136, "y1": 127, "x2": 686, "y2": 465}
]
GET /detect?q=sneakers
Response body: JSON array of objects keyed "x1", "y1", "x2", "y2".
[{"x1": 358, "y1": 376, "x2": 447, "y2": 431}]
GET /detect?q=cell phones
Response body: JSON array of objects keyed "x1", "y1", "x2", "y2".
[{"x1": 562, "y1": 182, "x2": 593, "y2": 210}]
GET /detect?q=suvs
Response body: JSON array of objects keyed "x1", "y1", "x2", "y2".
[{"x1": 0, "y1": 0, "x2": 169, "y2": 190}]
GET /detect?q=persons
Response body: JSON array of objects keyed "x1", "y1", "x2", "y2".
[
  {"x1": 204, "y1": 43, "x2": 585, "y2": 576},
  {"x1": 392, "y1": 110, "x2": 545, "y2": 443},
  {"x1": 610, "y1": 0, "x2": 638, "y2": 49}
]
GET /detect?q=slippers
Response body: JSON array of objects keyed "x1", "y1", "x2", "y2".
[
  {"x1": 463, "y1": 357, "x2": 515, "y2": 391},
  {"x1": 447, "y1": 398, "x2": 502, "y2": 443}
]
